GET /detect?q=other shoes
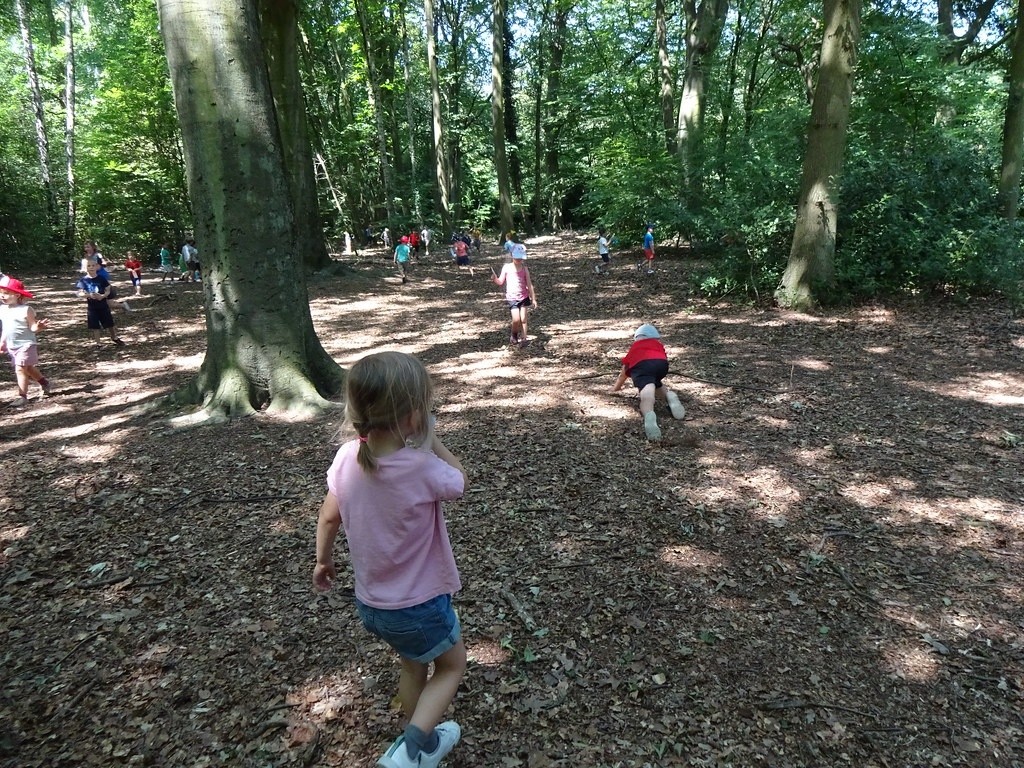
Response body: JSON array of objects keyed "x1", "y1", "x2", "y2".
[
  {"x1": 519, "y1": 337, "x2": 526, "y2": 346},
  {"x1": 402, "y1": 277, "x2": 406, "y2": 282},
  {"x1": 668, "y1": 391, "x2": 686, "y2": 420},
  {"x1": 10, "y1": 396, "x2": 29, "y2": 407},
  {"x1": 601, "y1": 272, "x2": 609, "y2": 275},
  {"x1": 646, "y1": 411, "x2": 662, "y2": 439},
  {"x1": 113, "y1": 338, "x2": 127, "y2": 347},
  {"x1": 596, "y1": 265, "x2": 600, "y2": 273},
  {"x1": 94, "y1": 345, "x2": 102, "y2": 357},
  {"x1": 648, "y1": 270, "x2": 654, "y2": 274},
  {"x1": 41, "y1": 384, "x2": 51, "y2": 400},
  {"x1": 456, "y1": 275, "x2": 462, "y2": 279},
  {"x1": 510, "y1": 336, "x2": 518, "y2": 345},
  {"x1": 638, "y1": 264, "x2": 642, "y2": 271}
]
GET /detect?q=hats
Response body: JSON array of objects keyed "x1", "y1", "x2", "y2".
[
  {"x1": 635, "y1": 325, "x2": 661, "y2": 338},
  {"x1": 0, "y1": 277, "x2": 33, "y2": 297},
  {"x1": 512, "y1": 245, "x2": 527, "y2": 259},
  {"x1": 401, "y1": 235, "x2": 409, "y2": 242}
]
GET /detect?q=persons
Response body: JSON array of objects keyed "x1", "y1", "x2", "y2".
[
  {"x1": 179, "y1": 239, "x2": 201, "y2": 283},
  {"x1": 408, "y1": 230, "x2": 421, "y2": 261},
  {"x1": 77, "y1": 239, "x2": 126, "y2": 347},
  {"x1": 313, "y1": 350, "x2": 468, "y2": 768},
  {"x1": 420, "y1": 225, "x2": 431, "y2": 255},
  {"x1": 161, "y1": 241, "x2": 178, "y2": 284},
  {"x1": 502, "y1": 233, "x2": 515, "y2": 255},
  {"x1": 394, "y1": 236, "x2": 410, "y2": 282},
  {"x1": 366, "y1": 225, "x2": 373, "y2": 244},
  {"x1": 491, "y1": 244, "x2": 537, "y2": 343},
  {"x1": 380, "y1": 228, "x2": 391, "y2": 248},
  {"x1": 608, "y1": 325, "x2": 685, "y2": 441},
  {"x1": 0, "y1": 276, "x2": 51, "y2": 406},
  {"x1": 125, "y1": 251, "x2": 143, "y2": 296},
  {"x1": 452, "y1": 226, "x2": 480, "y2": 278},
  {"x1": 595, "y1": 229, "x2": 612, "y2": 275},
  {"x1": 645, "y1": 225, "x2": 656, "y2": 273}
]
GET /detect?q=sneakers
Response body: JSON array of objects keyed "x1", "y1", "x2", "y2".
[
  {"x1": 375, "y1": 736, "x2": 419, "y2": 768},
  {"x1": 419, "y1": 721, "x2": 460, "y2": 768}
]
